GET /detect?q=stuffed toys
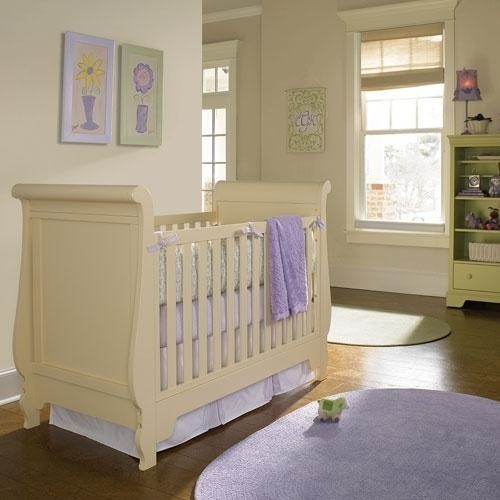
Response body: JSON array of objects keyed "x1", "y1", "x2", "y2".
[
  {"x1": 487, "y1": 174, "x2": 500, "y2": 197},
  {"x1": 464, "y1": 206, "x2": 500, "y2": 230},
  {"x1": 317, "y1": 396, "x2": 350, "y2": 423}
]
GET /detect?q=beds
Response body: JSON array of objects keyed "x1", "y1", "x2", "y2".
[{"x1": 10, "y1": 179, "x2": 334, "y2": 474}]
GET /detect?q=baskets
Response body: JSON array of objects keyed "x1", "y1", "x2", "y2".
[{"x1": 468, "y1": 241, "x2": 500, "y2": 263}]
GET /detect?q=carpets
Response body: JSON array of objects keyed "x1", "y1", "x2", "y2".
[
  {"x1": 192, "y1": 387, "x2": 499, "y2": 500},
  {"x1": 327, "y1": 302, "x2": 452, "y2": 347}
]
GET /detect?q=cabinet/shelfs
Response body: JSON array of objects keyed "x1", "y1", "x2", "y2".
[{"x1": 446, "y1": 134, "x2": 500, "y2": 308}]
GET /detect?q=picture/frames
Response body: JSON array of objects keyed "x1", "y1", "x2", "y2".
[{"x1": 59, "y1": 30, "x2": 165, "y2": 148}]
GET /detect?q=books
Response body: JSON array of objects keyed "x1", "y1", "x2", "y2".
[{"x1": 456, "y1": 188, "x2": 489, "y2": 198}]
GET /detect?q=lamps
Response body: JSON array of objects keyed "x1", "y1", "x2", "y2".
[{"x1": 452, "y1": 70, "x2": 483, "y2": 135}]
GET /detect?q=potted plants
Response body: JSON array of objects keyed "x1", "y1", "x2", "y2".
[{"x1": 464, "y1": 113, "x2": 492, "y2": 135}]
